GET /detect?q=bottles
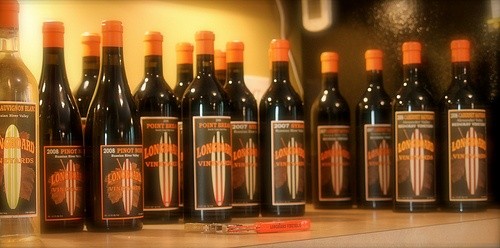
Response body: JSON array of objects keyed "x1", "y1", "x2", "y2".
[
  {"x1": 356, "y1": 46, "x2": 396, "y2": 210},
  {"x1": 392, "y1": 41, "x2": 439, "y2": 210},
  {"x1": 37, "y1": 21, "x2": 83, "y2": 233},
  {"x1": 437, "y1": 38, "x2": 490, "y2": 215},
  {"x1": 258, "y1": 39, "x2": 308, "y2": 216},
  {"x1": 310, "y1": 50, "x2": 355, "y2": 209},
  {"x1": 0, "y1": 0, "x2": 42, "y2": 244},
  {"x1": 171, "y1": 29, "x2": 260, "y2": 224},
  {"x1": 132, "y1": 31, "x2": 180, "y2": 224},
  {"x1": 74, "y1": 18, "x2": 145, "y2": 231}
]
